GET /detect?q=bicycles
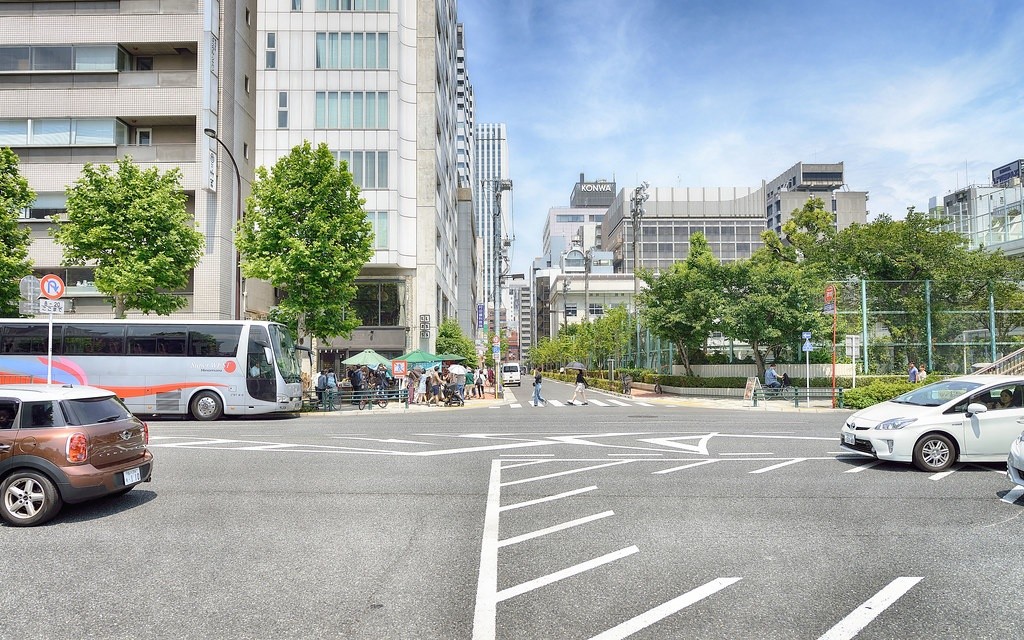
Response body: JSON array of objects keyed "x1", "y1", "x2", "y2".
[
  {"x1": 756, "y1": 377, "x2": 796, "y2": 402},
  {"x1": 359, "y1": 382, "x2": 389, "y2": 411},
  {"x1": 653, "y1": 376, "x2": 663, "y2": 394}
]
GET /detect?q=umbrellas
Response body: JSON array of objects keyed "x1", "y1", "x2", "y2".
[
  {"x1": 438, "y1": 352, "x2": 467, "y2": 360},
  {"x1": 448, "y1": 365, "x2": 467, "y2": 375},
  {"x1": 390, "y1": 348, "x2": 442, "y2": 362},
  {"x1": 341, "y1": 349, "x2": 390, "y2": 365},
  {"x1": 565, "y1": 362, "x2": 587, "y2": 377}
]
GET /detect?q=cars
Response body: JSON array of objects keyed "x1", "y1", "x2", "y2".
[{"x1": 839, "y1": 373, "x2": 1024, "y2": 489}]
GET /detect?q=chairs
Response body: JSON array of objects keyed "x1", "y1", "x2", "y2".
[{"x1": 1012, "y1": 387, "x2": 1022, "y2": 407}]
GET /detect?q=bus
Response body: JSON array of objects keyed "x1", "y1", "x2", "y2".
[{"x1": 0, "y1": 318, "x2": 314, "y2": 422}]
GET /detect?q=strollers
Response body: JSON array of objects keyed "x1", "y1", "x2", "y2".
[{"x1": 441, "y1": 383, "x2": 465, "y2": 407}]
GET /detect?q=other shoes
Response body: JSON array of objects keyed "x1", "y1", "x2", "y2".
[
  {"x1": 582, "y1": 403, "x2": 588, "y2": 405},
  {"x1": 426, "y1": 401, "x2": 430, "y2": 407},
  {"x1": 567, "y1": 401, "x2": 573, "y2": 405}
]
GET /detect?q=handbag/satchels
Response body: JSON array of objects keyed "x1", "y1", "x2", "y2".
[{"x1": 783, "y1": 373, "x2": 790, "y2": 385}]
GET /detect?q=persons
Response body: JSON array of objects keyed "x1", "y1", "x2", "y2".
[
  {"x1": 765, "y1": 364, "x2": 784, "y2": 395},
  {"x1": 488, "y1": 367, "x2": 494, "y2": 386},
  {"x1": 251, "y1": 360, "x2": 267, "y2": 378},
  {"x1": 407, "y1": 368, "x2": 428, "y2": 405},
  {"x1": 909, "y1": 361, "x2": 926, "y2": 383},
  {"x1": 443, "y1": 366, "x2": 486, "y2": 399},
  {"x1": 533, "y1": 369, "x2": 547, "y2": 407},
  {"x1": 978, "y1": 389, "x2": 1015, "y2": 410},
  {"x1": 567, "y1": 370, "x2": 588, "y2": 405},
  {"x1": 427, "y1": 366, "x2": 450, "y2": 407},
  {"x1": 346, "y1": 365, "x2": 391, "y2": 405},
  {"x1": 560, "y1": 366, "x2": 564, "y2": 374},
  {"x1": 314, "y1": 368, "x2": 338, "y2": 403}
]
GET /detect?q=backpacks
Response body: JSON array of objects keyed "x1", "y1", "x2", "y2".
[{"x1": 351, "y1": 371, "x2": 360, "y2": 386}]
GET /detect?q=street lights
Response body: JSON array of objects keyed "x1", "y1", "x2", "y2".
[{"x1": 204, "y1": 127, "x2": 242, "y2": 321}]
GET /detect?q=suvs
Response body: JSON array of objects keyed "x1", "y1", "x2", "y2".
[{"x1": 0, "y1": 383, "x2": 155, "y2": 527}]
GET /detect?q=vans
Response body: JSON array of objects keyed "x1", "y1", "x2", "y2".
[{"x1": 501, "y1": 363, "x2": 522, "y2": 387}]
